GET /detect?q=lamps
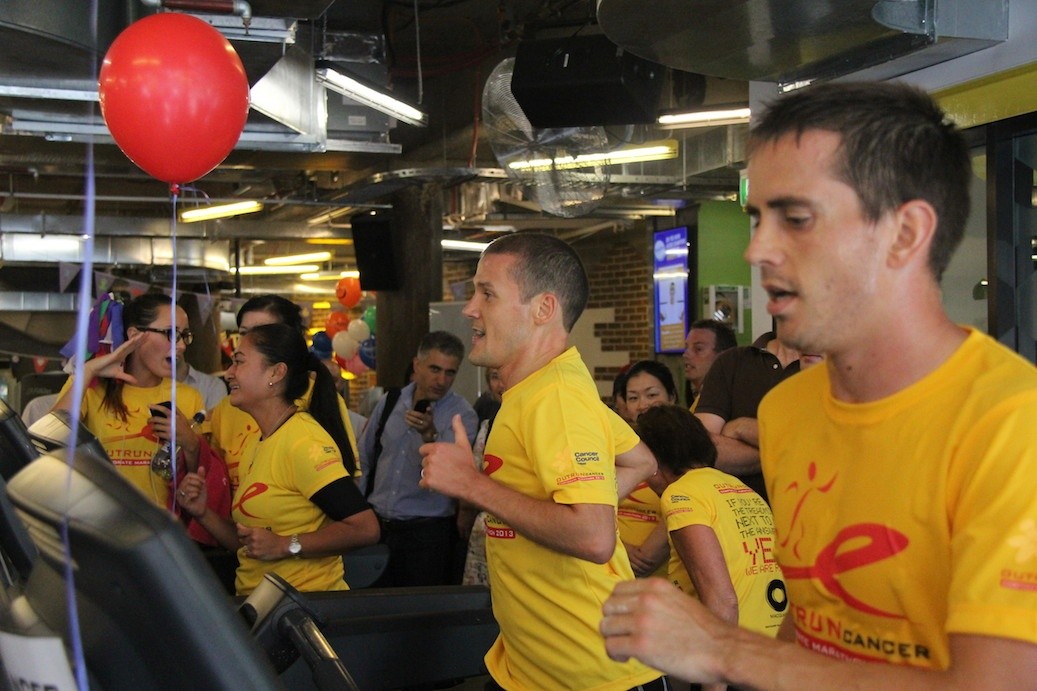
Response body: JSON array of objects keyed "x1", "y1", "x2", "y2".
[
  {"x1": 508, "y1": 137, "x2": 678, "y2": 172},
  {"x1": 179, "y1": 13, "x2": 299, "y2": 46},
  {"x1": 314, "y1": 0, "x2": 430, "y2": 128},
  {"x1": 653, "y1": 99, "x2": 752, "y2": 129},
  {"x1": 179, "y1": 200, "x2": 265, "y2": 223}
]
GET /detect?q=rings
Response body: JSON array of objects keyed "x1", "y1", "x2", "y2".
[
  {"x1": 420, "y1": 468, "x2": 425, "y2": 477},
  {"x1": 420, "y1": 420, "x2": 424, "y2": 425},
  {"x1": 179, "y1": 489, "x2": 188, "y2": 501}
]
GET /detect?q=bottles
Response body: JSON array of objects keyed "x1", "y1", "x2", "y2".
[{"x1": 151, "y1": 410, "x2": 208, "y2": 481}]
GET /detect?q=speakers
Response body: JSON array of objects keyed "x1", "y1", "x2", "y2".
[
  {"x1": 511, "y1": 33, "x2": 664, "y2": 129},
  {"x1": 350, "y1": 207, "x2": 402, "y2": 293}
]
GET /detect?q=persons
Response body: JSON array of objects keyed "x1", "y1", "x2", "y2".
[
  {"x1": 355, "y1": 330, "x2": 480, "y2": 586},
  {"x1": 175, "y1": 322, "x2": 382, "y2": 598},
  {"x1": 159, "y1": 345, "x2": 228, "y2": 413},
  {"x1": 682, "y1": 318, "x2": 738, "y2": 423},
  {"x1": 631, "y1": 399, "x2": 795, "y2": 690},
  {"x1": 796, "y1": 341, "x2": 827, "y2": 374},
  {"x1": 50, "y1": 289, "x2": 212, "y2": 523},
  {"x1": 212, "y1": 292, "x2": 361, "y2": 548},
  {"x1": 470, "y1": 363, "x2": 505, "y2": 422},
  {"x1": 613, "y1": 362, "x2": 634, "y2": 425},
  {"x1": 419, "y1": 229, "x2": 667, "y2": 691},
  {"x1": 452, "y1": 414, "x2": 500, "y2": 589},
  {"x1": 690, "y1": 335, "x2": 801, "y2": 521},
  {"x1": 598, "y1": 78, "x2": 1036, "y2": 691},
  {"x1": 605, "y1": 357, "x2": 700, "y2": 599}
]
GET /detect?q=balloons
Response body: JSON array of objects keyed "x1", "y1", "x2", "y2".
[
  {"x1": 98, "y1": 13, "x2": 249, "y2": 197},
  {"x1": 304, "y1": 277, "x2": 379, "y2": 383}
]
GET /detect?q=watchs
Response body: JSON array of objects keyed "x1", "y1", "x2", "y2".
[
  {"x1": 288, "y1": 531, "x2": 301, "y2": 556},
  {"x1": 425, "y1": 431, "x2": 440, "y2": 442}
]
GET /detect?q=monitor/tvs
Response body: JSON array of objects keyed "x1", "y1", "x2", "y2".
[{"x1": 653, "y1": 225, "x2": 694, "y2": 358}]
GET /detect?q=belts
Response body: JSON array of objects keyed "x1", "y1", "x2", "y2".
[{"x1": 380, "y1": 517, "x2": 445, "y2": 529}]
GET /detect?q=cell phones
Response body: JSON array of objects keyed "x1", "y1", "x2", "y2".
[{"x1": 414, "y1": 399, "x2": 429, "y2": 414}]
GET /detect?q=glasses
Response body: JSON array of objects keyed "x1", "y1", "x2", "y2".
[{"x1": 135, "y1": 325, "x2": 195, "y2": 345}]
